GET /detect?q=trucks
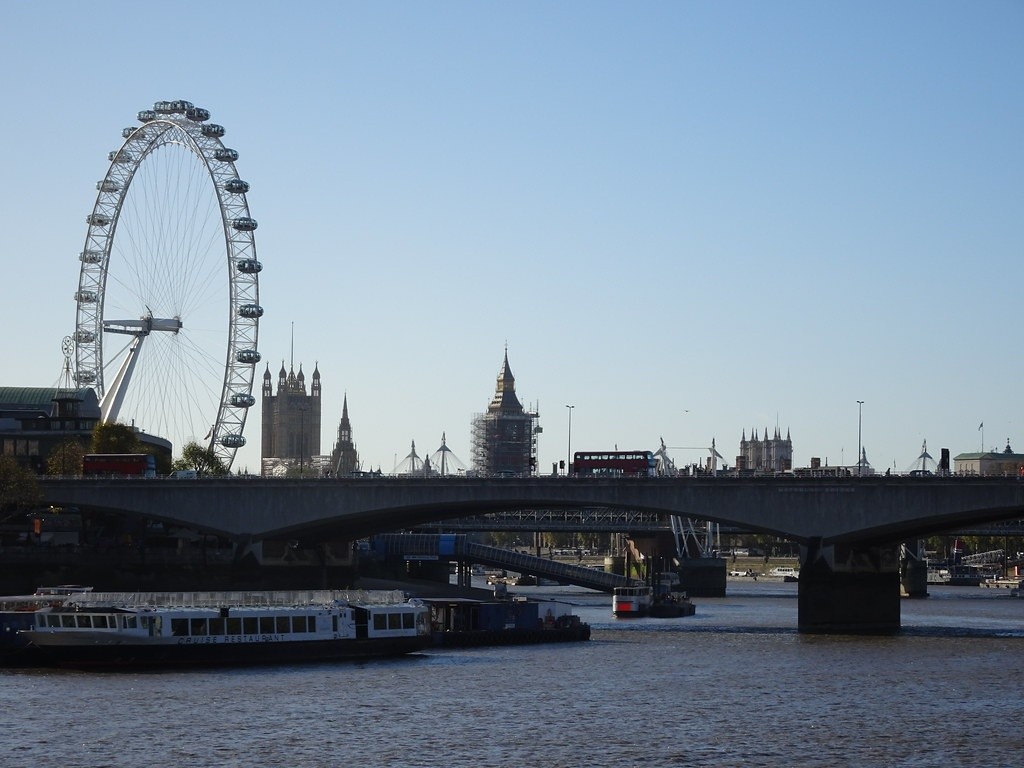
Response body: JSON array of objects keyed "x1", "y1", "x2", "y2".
[{"x1": 167, "y1": 471, "x2": 198, "y2": 479}]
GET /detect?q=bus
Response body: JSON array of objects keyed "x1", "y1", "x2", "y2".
[
  {"x1": 83, "y1": 454, "x2": 148, "y2": 476},
  {"x1": 574, "y1": 452, "x2": 656, "y2": 477}
]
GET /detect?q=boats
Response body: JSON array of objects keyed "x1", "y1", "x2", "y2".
[
  {"x1": 612, "y1": 587, "x2": 653, "y2": 618},
  {"x1": 0, "y1": 585, "x2": 94, "y2": 645},
  {"x1": 16, "y1": 596, "x2": 439, "y2": 679},
  {"x1": 648, "y1": 593, "x2": 696, "y2": 618},
  {"x1": 417, "y1": 599, "x2": 591, "y2": 648},
  {"x1": 942, "y1": 565, "x2": 986, "y2": 586}
]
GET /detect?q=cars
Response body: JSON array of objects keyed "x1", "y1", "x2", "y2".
[
  {"x1": 731, "y1": 549, "x2": 748, "y2": 556},
  {"x1": 493, "y1": 470, "x2": 518, "y2": 477}
]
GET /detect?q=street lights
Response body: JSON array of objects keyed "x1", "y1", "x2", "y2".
[
  {"x1": 38, "y1": 416, "x2": 65, "y2": 474},
  {"x1": 857, "y1": 401, "x2": 864, "y2": 476},
  {"x1": 298, "y1": 407, "x2": 307, "y2": 479},
  {"x1": 566, "y1": 405, "x2": 574, "y2": 473}
]
